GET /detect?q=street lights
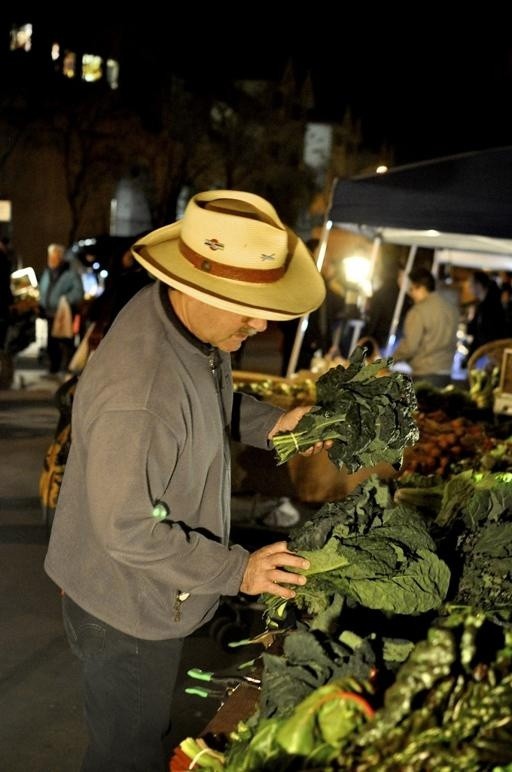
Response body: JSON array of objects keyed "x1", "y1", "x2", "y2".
[
  {"x1": 325, "y1": 160, "x2": 388, "y2": 221},
  {"x1": 342, "y1": 251, "x2": 374, "y2": 355}
]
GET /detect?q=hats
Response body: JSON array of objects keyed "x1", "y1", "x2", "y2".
[{"x1": 126, "y1": 187, "x2": 332, "y2": 324}]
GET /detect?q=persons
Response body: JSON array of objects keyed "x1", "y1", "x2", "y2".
[
  {"x1": 1, "y1": 222, "x2": 157, "y2": 390},
  {"x1": 43, "y1": 189, "x2": 334, "y2": 772},
  {"x1": 279, "y1": 238, "x2": 509, "y2": 389}
]
